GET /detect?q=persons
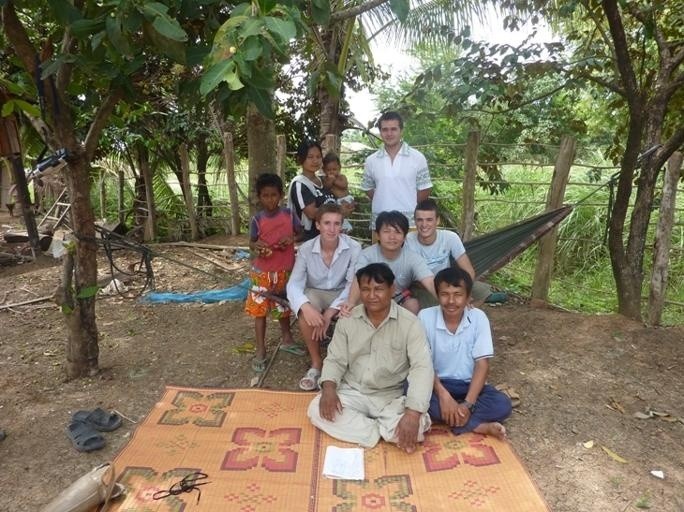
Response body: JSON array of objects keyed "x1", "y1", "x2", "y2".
[
  {"x1": 335, "y1": 209, "x2": 475, "y2": 319},
  {"x1": 321, "y1": 151, "x2": 355, "y2": 234},
  {"x1": 360, "y1": 111, "x2": 434, "y2": 245},
  {"x1": 286, "y1": 139, "x2": 357, "y2": 248},
  {"x1": 403, "y1": 198, "x2": 476, "y2": 311},
  {"x1": 403, "y1": 266, "x2": 512, "y2": 442},
  {"x1": 243, "y1": 172, "x2": 306, "y2": 373},
  {"x1": 285, "y1": 202, "x2": 362, "y2": 392},
  {"x1": 306, "y1": 262, "x2": 434, "y2": 454}
]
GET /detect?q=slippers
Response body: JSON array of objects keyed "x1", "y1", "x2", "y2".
[
  {"x1": 61, "y1": 423, "x2": 106, "y2": 452},
  {"x1": 277, "y1": 342, "x2": 307, "y2": 359},
  {"x1": 69, "y1": 408, "x2": 123, "y2": 431},
  {"x1": 250, "y1": 349, "x2": 268, "y2": 373},
  {"x1": 298, "y1": 367, "x2": 320, "y2": 390}
]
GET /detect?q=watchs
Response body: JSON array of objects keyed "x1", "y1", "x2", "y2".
[{"x1": 462, "y1": 399, "x2": 476, "y2": 415}]
